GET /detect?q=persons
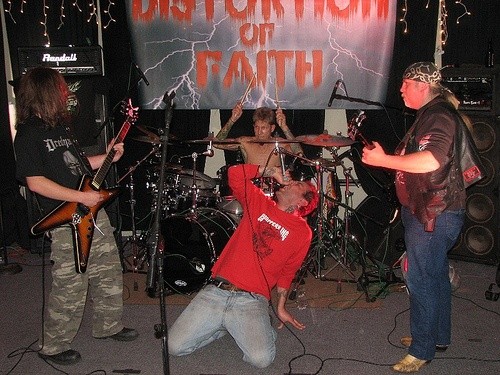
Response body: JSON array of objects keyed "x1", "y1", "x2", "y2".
[
  {"x1": 361, "y1": 62, "x2": 466, "y2": 373},
  {"x1": 161, "y1": 164, "x2": 320, "y2": 368},
  {"x1": 13, "y1": 66, "x2": 139, "y2": 367},
  {"x1": 212, "y1": 102, "x2": 306, "y2": 192}
]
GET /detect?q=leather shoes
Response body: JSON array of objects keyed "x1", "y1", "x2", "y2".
[
  {"x1": 39, "y1": 348, "x2": 82, "y2": 365},
  {"x1": 94, "y1": 327, "x2": 139, "y2": 341}
]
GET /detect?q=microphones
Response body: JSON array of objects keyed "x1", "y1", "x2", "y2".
[
  {"x1": 132, "y1": 61, "x2": 149, "y2": 85},
  {"x1": 279, "y1": 154, "x2": 288, "y2": 182},
  {"x1": 328, "y1": 81, "x2": 340, "y2": 107}
]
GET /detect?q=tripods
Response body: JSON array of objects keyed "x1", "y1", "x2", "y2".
[
  {"x1": 117, "y1": 144, "x2": 161, "y2": 291},
  {"x1": 290, "y1": 147, "x2": 405, "y2": 301}
]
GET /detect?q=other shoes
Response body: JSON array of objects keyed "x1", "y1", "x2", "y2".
[
  {"x1": 400, "y1": 338, "x2": 448, "y2": 353},
  {"x1": 389, "y1": 354, "x2": 433, "y2": 374}
]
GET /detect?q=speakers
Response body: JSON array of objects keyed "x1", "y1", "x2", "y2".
[
  {"x1": 58, "y1": 73, "x2": 107, "y2": 156},
  {"x1": 343, "y1": 195, "x2": 407, "y2": 266},
  {"x1": 446, "y1": 115, "x2": 500, "y2": 267}
]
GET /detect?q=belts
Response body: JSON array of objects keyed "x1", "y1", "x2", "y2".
[{"x1": 208, "y1": 278, "x2": 250, "y2": 292}]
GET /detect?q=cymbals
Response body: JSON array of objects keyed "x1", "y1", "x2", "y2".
[
  {"x1": 296, "y1": 134, "x2": 356, "y2": 147},
  {"x1": 246, "y1": 138, "x2": 303, "y2": 144},
  {"x1": 180, "y1": 138, "x2": 242, "y2": 145},
  {"x1": 130, "y1": 122, "x2": 182, "y2": 146},
  {"x1": 302, "y1": 159, "x2": 340, "y2": 167}
]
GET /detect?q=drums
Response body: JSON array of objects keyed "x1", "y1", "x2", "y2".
[
  {"x1": 145, "y1": 207, "x2": 239, "y2": 296},
  {"x1": 216, "y1": 196, "x2": 243, "y2": 226},
  {"x1": 166, "y1": 169, "x2": 218, "y2": 216},
  {"x1": 304, "y1": 170, "x2": 341, "y2": 215}
]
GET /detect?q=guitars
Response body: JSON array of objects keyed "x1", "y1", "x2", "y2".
[
  {"x1": 30, "y1": 101, "x2": 139, "y2": 275},
  {"x1": 348, "y1": 111, "x2": 402, "y2": 212}
]
